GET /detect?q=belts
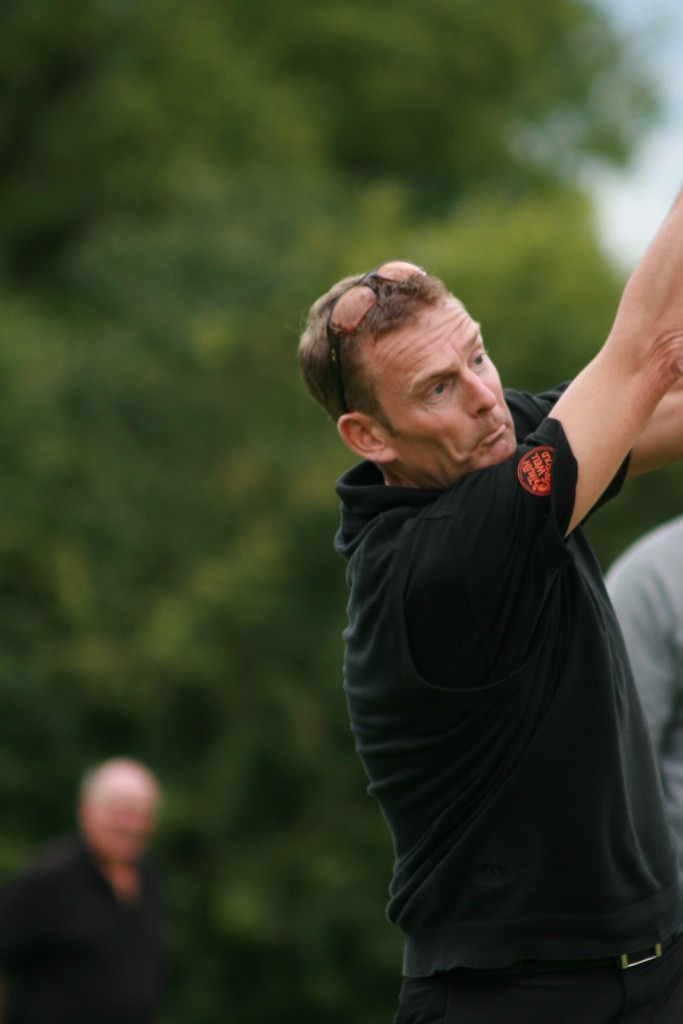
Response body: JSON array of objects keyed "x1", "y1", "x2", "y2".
[{"x1": 438, "y1": 932, "x2": 683, "y2": 979}]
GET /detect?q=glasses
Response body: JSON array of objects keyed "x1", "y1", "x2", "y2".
[{"x1": 327, "y1": 260, "x2": 419, "y2": 417}]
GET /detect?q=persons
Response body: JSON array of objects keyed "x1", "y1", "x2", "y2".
[
  {"x1": 298, "y1": 184, "x2": 683, "y2": 1024},
  {"x1": 0, "y1": 758, "x2": 167, "y2": 1024}
]
